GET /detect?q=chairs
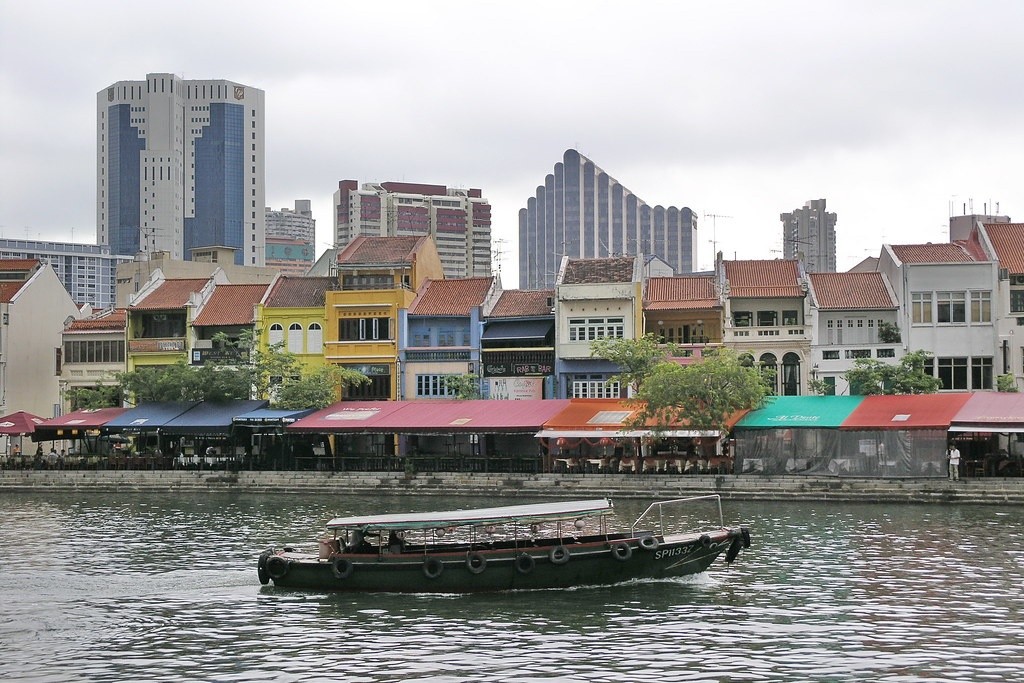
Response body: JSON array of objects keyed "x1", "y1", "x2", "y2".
[
  {"x1": 0, "y1": 449, "x2": 240, "y2": 470},
  {"x1": 339, "y1": 537, "x2": 352, "y2": 554},
  {"x1": 550, "y1": 453, "x2": 1023, "y2": 477}
]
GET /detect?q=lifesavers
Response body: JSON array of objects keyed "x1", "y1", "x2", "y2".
[
  {"x1": 548, "y1": 545, "x2": 572, "y2": 566},
  {"x1": 463, "y1": 551, "x2": 487, "y2": 576},
  {"x1": 256, "y1": 551, "x2": 290, "y2": 586},
  {"x1": 329, "y1": 556, "x2": 355, "y2": 582},
  {"x1": 610, "y1": 541, "x2": 633, "y2": 563},
  {"x1": 636, "y1": 534, "x2": 660, "y2": 554},
  {"x1": 420, "y1": 556, "x2": 445, "y2": 581},
  {"x1": 513, "y1": 553, "x2": 537, "y2": 576}
]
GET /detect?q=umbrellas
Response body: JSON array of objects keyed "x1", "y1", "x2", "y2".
[{"x1": 0, "y1": 410, "x2": 48, "y2": 457}]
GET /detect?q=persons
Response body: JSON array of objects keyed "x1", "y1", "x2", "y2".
[
  {"x1": 350, "y1": 526, "x2": 383, "y2": 554},
  {"x1": 13, "y1": 444, "x2": 21, "y2": 457},
  {"x1": 35, "y1": 442, "x2": 45, "y2": 456},
  {"x1": 47, "y1": 448, "x2": 66, "y2": 470},
  {"x1": 948, "y1": 444, "x2": 961, "y2": 481}
]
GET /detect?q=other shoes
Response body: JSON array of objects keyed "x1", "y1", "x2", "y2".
[{"x1": 948, "y1": 478, "x2": 960, "y2": 482}]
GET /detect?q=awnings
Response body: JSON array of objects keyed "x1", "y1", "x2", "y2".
[
  {"x1": 947, "y1": 391, "x2": 1024, "y2": 433},
  {"x1": 33, "y1": 406, "x2": 132, "y2": 431},
  {"x1": 367, "y1": 399, "x2": 571, "y2": 435},
  {"x1": 839, "y1": 392, "x2": 974, "y2": 430},
  {"x1": 233, "y1": 407, "x2": 320, "y2": 428},
  {"x1": 733, "y1": 395, "x2": 868, "y2": 430},
  {"x1": 163, "y1": 398, "x2": 270, "y2": 437},
  {"x1": 533, "y1": 398, "x2": 750, "y2": 438},
  {"x1": 102, "y1": 401, "x2": 201, "y2": 431},
  {"x1": 286, "y1": 400, "x2": 412, "y2": 435}
]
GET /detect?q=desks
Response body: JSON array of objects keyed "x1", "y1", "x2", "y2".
[
  {"x1": 684, "y1": 459, "x2": 707, "y2": 472},
  {"x1": 587, "y1": 458, "x2": 605, "y2": 469},
  {"x1": 878, "y1": 461, "x2": 895, "y2": 467},
  {"x1": 708, "y1": 458, "x2": 733, "y2": 470},
  {"x1": 921, "y1": 460, "x2": 941, "y2": 473},
  {"x1": 742, "y1": 458, "x2": 776, "y2": 472},
  {"x1": 785, "y1": 458, "x2": 807, "y2": 473},
  {"x1": 619, "y1": 458, "x2": 636, "y2": 471},
  {"x1": 555, "y1": 458, "x2": 577, "y2": 468},
  {"x1": 828, "y1": 458, "x2": 859, "y2": 473},
  {"x1": 664, "y1": 460, "x2": 685, "y2": 473},
  {"x1": 642, "y1": 459, "x2": 662, "y2": 472}
]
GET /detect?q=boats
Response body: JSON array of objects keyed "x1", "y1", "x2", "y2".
[{"x1": 257, "y1": 494, "x2": 751, "y2": 592}]
